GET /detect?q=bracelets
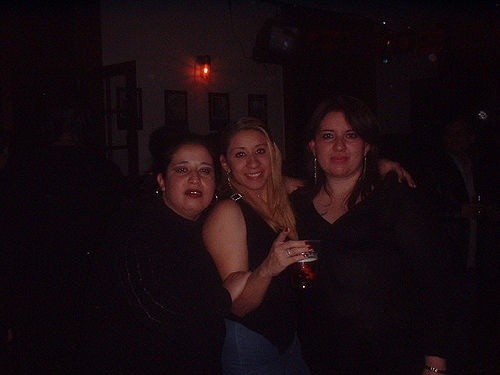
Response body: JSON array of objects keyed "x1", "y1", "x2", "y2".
[{"x1": 425, "y1": 365, "x2": 448, "y2": 374}]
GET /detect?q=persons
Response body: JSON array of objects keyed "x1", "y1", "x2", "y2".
[
  {"x1": 84, "y1": 137, "x2": 252, "y2": 375},
  {"x1": 201, "y1": 116, "x2": 417, "y2": 375},
  {"x1": 288, "y1": 96, "x2": 457, "y2": 375}
]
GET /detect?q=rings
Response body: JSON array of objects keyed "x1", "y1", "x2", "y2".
[{"x1": 286, "y1": 249, "x2": 291, "y2": 256}]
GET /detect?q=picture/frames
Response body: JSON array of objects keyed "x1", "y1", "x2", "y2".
[
  {"x1": 163, "y1": 89, "x2": 188, "y2": 128},
  {"x1": 247, "y1": 94, "x2": 268, "y2": 125},
  {"x1": 117, "y1": 87, "x2": 143, "y2": 130},
  {"x1": 208, "y1": 92, "x2": 230, "y2": 132}
]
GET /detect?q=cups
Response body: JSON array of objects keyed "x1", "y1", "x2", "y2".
[{"x1": 286, "y1": 240, "x2": 320, "y2": 293}]
